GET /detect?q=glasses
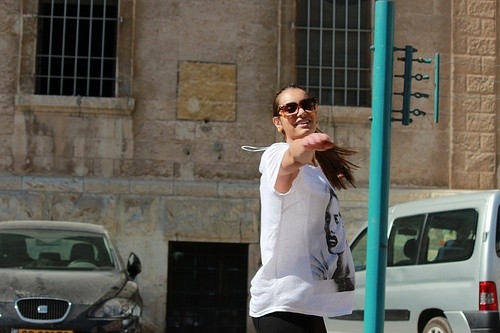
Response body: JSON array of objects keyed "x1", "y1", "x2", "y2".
[{"x1": 276, "y1": 97, "x2": 317, "y2": 116}]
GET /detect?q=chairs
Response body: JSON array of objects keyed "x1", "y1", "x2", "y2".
[
  {"x1": 392, "y1": 238, "x2": 419, "y2": 267},
  {"x1": 0, "y1": 235, "x2": 30, "y2": 267},
  {"x1": 70, "y1": 243, "x2": 102, "y2": 266}
]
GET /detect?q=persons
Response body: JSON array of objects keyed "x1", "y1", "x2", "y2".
[{"x1": 247, "y1": 84, "x2": 357, "y2": 333}]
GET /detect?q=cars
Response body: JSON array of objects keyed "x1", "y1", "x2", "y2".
[{"x1": 0, "y1": 221, "x2": 142, "y2": 332}]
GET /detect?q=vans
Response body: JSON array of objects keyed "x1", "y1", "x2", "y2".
[{"x1": 325, "y1": 188, "x2": 498, "y2": 332}]
